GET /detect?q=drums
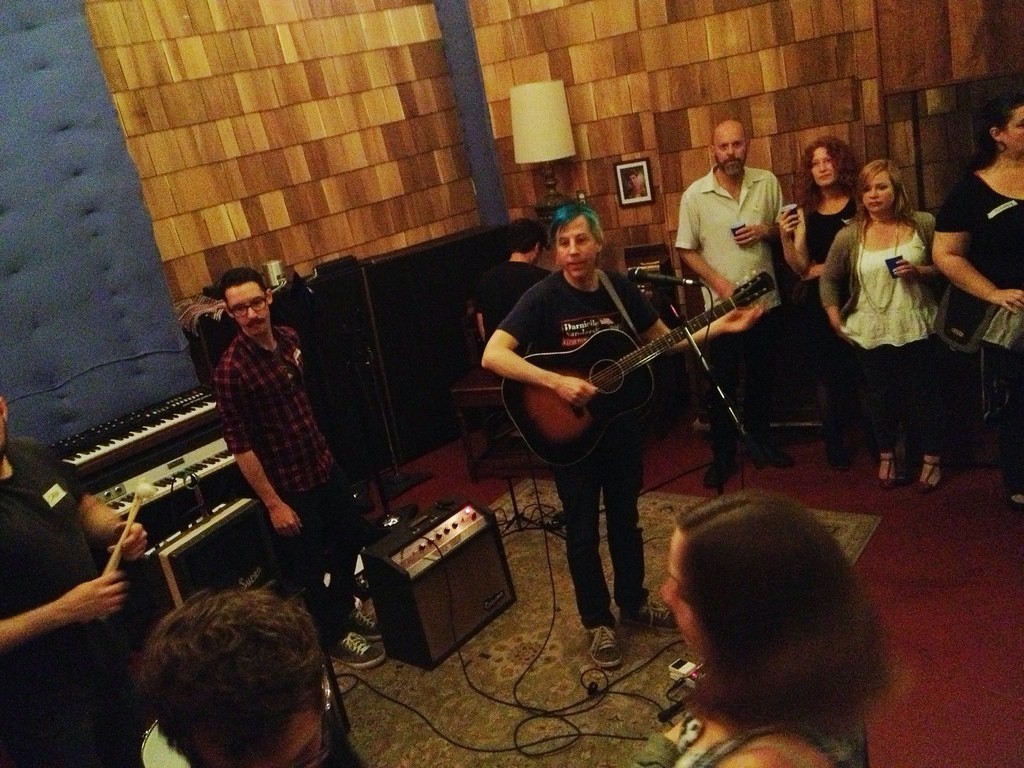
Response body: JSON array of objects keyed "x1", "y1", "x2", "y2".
[{"x1": 140, "y1": 663, "x2": 332, "y2": 768}]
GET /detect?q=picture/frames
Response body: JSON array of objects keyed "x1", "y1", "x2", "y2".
[{"x1": 612, "y1": 156, "x2": 656, "y2": 208}]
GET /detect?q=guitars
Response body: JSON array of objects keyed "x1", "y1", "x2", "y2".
[{"x1": 500, "y1": 267, "x2": 776, "y2": 470}]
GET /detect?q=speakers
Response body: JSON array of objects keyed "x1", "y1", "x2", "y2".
[{"x1": 371, "y1": 494, "x2": 518, "y2": 672}]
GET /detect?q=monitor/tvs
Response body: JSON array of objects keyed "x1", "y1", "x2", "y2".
[{"x1": 148, "y1": 498, "x2": 280, "y2": 619}]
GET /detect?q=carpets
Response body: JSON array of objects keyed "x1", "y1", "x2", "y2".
[{"x1": 328, "y1": 475, "x2": 890, "y2": 768}]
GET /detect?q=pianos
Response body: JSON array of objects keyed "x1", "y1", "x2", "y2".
[{"x1": 49, "y1": 384, "x2": 237, "y2": 518}]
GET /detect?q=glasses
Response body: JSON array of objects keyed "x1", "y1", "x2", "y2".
[{"x1": 225, "y1": 291, "x2": 266, "y2": 318}]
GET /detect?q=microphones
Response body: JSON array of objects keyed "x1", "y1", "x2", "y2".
[{"x1": 627, "y1": 268, "x2": 699, "y2": 288}]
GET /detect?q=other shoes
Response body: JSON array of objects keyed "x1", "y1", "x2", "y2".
[
  {"x1": 877, "y1": 451, "x2": 897, "y2": 488},
  {"x1": 824, "y1": 430, "x2": 843, "y2": 465},
  {"x1": 867, "y1": 433, "x2": 880, "y2": 461},
  {"x1": 1006, "y1": 482, "x2": 1024, "y2": 510},
  {"x1": 703, "y1": 451, "x2": 737, "y2": 486},
  {"x1": 920, "y1": 454, "x2": 943, "y2": 488},
  {"x1": 750, "y1": 444, "x2": 795, "y2": 470}
]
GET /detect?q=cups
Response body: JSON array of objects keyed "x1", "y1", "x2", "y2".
[
  {"x1": 731, "y1": 222, "x2": 745, "y2": 237},
  {"x1": 780, "y1": 204, "x2": 799, "y2": 228},
  {"x1": 885, "y1": 255, "x2": 903, "y2": 279}
]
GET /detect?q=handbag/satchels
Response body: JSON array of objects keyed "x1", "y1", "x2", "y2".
[{"x1": 932, "y1": 282, "x2": 999, "y2": 354}]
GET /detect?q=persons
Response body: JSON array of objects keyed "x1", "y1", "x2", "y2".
[
  {"x1": 628, "y1": 489, "x2": 890, "y2": 768},
  {"x1": 208, "y1": 267, "x2": 386, "y2": 672},
  {"x1": 624, "y1": 167, "x2": 647, "y2": 199},
  {"x1": 480, "y1": 203, "x2": 763, "y2": 674},
  {"x1": 0, "y1": 392, "x2": 148, "y2": 768},
  {"x1": 671, "y1": 120, "x2": 795, "y2": 487},
  {"x1": 776, "y1": 135, "x2": 884, "y2": 472},
  {"x1": 475, "y1": 218, "x2": 552, "y2": 350},
  {"x1": 120, "y1": 587, "x2": 364, "y2": 768},
  {"x1": 931, "y1": 89, "x2": 1024, "y2": 510},
  {"x1": 818, "y1": 160, "x2": 950, "y2": 496}
]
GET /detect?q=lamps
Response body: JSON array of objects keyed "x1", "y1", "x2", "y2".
[{"x1": 509, "y1": 78, "x2": 577, "y2": 225}]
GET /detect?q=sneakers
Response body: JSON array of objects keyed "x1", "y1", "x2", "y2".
[
  {"x1": 345, "y1": 607, "x2": 382, "y2": 641},
  {"x1": 587, "y1": 625, "x2": 623, "y2": 668},
  {"x1": 619, "y1": 598, "x2": 681, "y2": 632},
  {"x1": 330, "y1": 631, "x2": 386, "y2": 669}
]
